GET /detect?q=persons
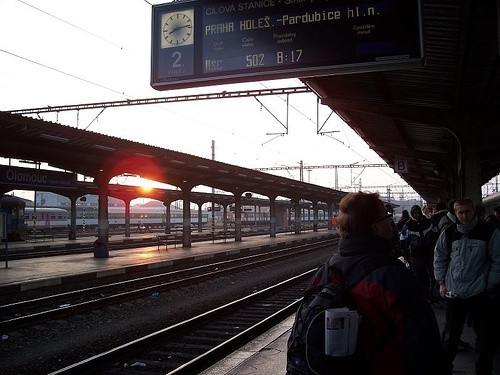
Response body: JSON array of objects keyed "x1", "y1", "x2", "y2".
[
  {"x1": 306, "y1": 193, "x2": 454, "y2": 375},
  {"x1": 383, "y1": 200, "x2": 500, "y2": 349},
  {"x1": 332, "y1": 215, "x2": 339, "y2": 229},
  {"x1": 433, "y1": 199, "x2": 500, "y2": 375}
]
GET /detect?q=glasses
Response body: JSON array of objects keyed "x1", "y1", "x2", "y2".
[{"x1": 372, "y1": 207, "x2": 396, "y2": 224}]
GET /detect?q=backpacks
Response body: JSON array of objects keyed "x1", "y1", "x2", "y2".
[{"x1": 285, "y1": 255, "x2": 397, "y2": 375}]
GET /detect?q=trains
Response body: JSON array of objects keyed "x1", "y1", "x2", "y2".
[
  {"x1": 482, "y1": 191, "x2": 500, "y2": 222},
  {"x1": 206, "y1": 205, "x2": 286, "y2": 223},
  {"x1": 23, "y1": 206, "x2": 208, "y2": 231}
]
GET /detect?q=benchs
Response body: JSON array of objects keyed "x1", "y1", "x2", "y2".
[
  {"x1": 283, "y1": 226, "x2": 295, "y2": 235},
  {"x1": 26, "y1": 228, "x2": 54, "y2": 242},
  {"x1": 154, "y1": 234, "x2": 182, "y2": 251},
  {"x1": 213, "y1": 230, "x2": 235, "y2": 244},
  {"x1": 301, "y1": 225, "x2": 312, "y2": 233}
]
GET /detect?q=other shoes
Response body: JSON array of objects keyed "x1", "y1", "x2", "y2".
[{"x1": 457, "y1": 339, "x2": 469, "y2": 351}]
